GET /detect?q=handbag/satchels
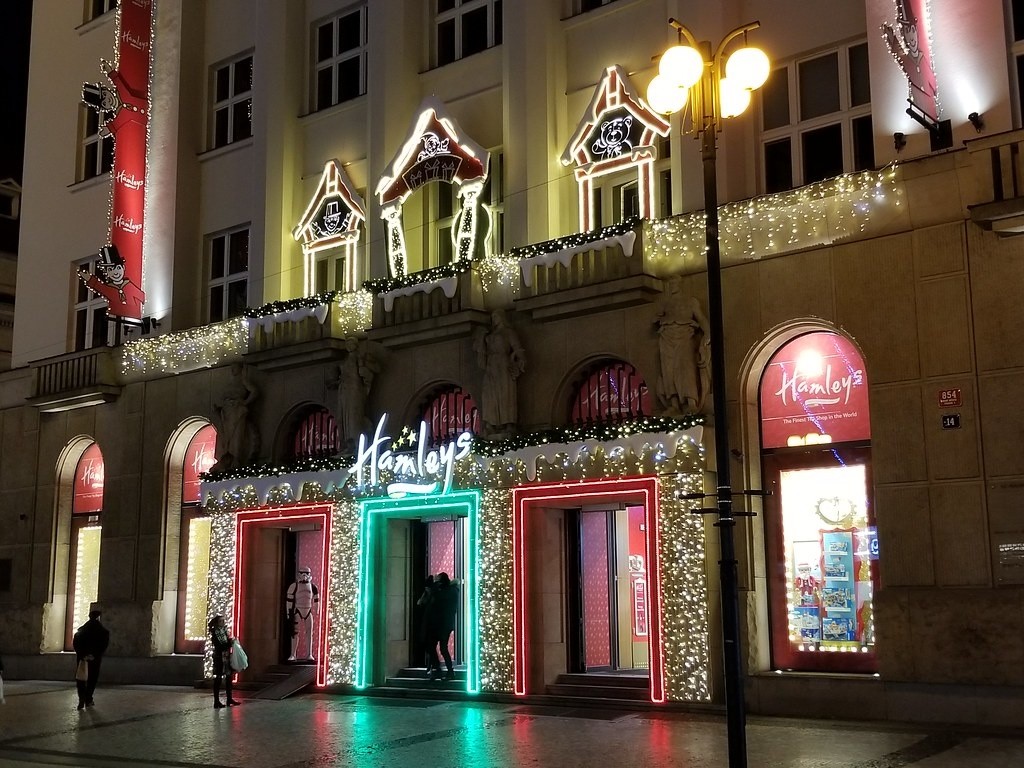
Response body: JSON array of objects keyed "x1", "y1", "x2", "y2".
[
  {"x1": 231, "y1": 641, "x2": 248, "y2": 671},
  {"x1": 74, "y1": 660, "x2": 88, "y2": 681}
]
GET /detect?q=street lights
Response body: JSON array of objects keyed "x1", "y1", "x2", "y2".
[{"x1": 647, "y1": 16, "x2": 771, "y2": 768}]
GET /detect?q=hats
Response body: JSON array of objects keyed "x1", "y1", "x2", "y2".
[
  {"x1": 424, "y1": 575, "x2": 434, "y2": 587},
  {"x1": 89, "y1": 611, "x2": 101, "y2": 617}
]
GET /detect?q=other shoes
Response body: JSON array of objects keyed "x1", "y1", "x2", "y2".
[
  {"x1": 214, "y1": 701, "x2": 226, "y2": 708},
  {"x1": 227, "y1": 699, "x2": 240, "y2": 705},
  {"x1": 77, "y1": 702, "x2": 95, "y2": 709},
  {"x1": 424, "y1": 668, "x2": 456, "y2": 680}
]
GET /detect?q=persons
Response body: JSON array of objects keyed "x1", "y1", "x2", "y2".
[
  {"x1": 435, "y1": 572, "x2": 461, "y2": 681},
  {"x1": 208, "y1": 616, "x2": 249, "y2": 708},
  {"x1": 73, "y1": 610, "x2": 109, "y2": 708},
  {"x1": 417, "y1": 574, "x2": 448, "y2": 680},
  {"x1": 287, "y1": 566, "x2": 319, "y2": 660}
]
